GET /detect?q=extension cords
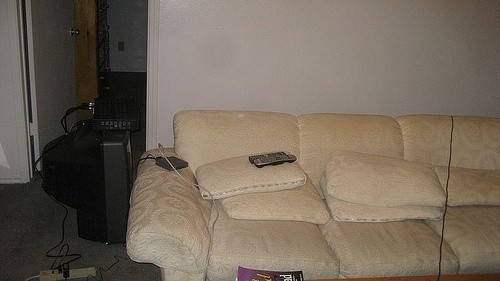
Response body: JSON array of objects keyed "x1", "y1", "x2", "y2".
[{"x1": 40, "y1": 265, "x2": 98, "y2": 281}]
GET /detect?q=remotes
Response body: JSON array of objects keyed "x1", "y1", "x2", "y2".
[
  {"x1": 249, "y1": 151, "x2": 287, "y2": 163},
  {"x1": 254, "y1": 155, "x2": 297, "y2": 167}
]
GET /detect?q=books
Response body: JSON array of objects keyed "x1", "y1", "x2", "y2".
[{"x1": 237, "y1": 266, "x2": 304, "y2": 281}]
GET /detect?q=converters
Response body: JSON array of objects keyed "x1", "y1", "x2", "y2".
[{"x1": 63, "y1": 262, "x2": 70, "y2": 277}]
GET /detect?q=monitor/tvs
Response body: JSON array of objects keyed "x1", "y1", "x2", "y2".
[{"x1": 40, "y1": 131, "x2": 134, "y2": 245}]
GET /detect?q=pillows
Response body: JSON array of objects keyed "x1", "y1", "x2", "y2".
[
  {"x1": 325, "y1": 151, "x2": 447, "y2": 208},
  {"x1": 219, "y1": 175, "x2": 330, "y2": 225},
  {"x1": 432, "y1": 164, "x2": 500, "y2": 206},
  {"x1": 319, "y1": 169, "x2": 443, "y2": 223},
  {"x1": 195, "y1": 152, "x2": 307, "y2": 199}
]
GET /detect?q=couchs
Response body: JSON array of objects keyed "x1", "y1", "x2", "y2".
[{"x1": 126, "y1": 110, "x2": 500, "y2": 281}]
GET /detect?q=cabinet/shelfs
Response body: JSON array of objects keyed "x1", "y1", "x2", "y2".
[{"x1": 75, "y1": 0, "x2": 110, "y2": 118}]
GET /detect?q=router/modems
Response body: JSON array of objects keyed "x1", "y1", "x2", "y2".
[{"x1": 157, "y1": 155, "x2": 190, "y2": 172}]
